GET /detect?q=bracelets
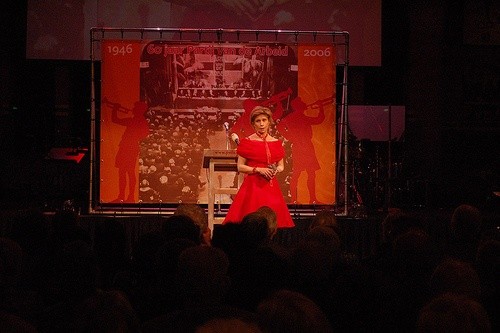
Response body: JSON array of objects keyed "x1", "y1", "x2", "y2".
[
  {"x1": 276, "y1": 168, "x2": 279, "y2": 173},
  {"x1": 253, "y1": 167, "x2": 256, "y2": 175}
]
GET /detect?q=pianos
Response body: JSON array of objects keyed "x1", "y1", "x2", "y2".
[{"x1": 201, "y1": 145, "x2": 241, "y2": 174}]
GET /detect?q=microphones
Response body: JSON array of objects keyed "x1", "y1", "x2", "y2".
[{"x1": 224, "y1": 122, "x2": 229, "y2": 129}]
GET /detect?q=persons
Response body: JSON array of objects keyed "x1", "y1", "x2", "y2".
[
  {"x1": 222, "y1": 105, "x2": 294, "y2": 228},
  {"x1": 133, "y1": 45, "x2": 293, "y2": 201},
  {"x1": 0, "y1": 201, "x2": 499, "y2": 333}
]
